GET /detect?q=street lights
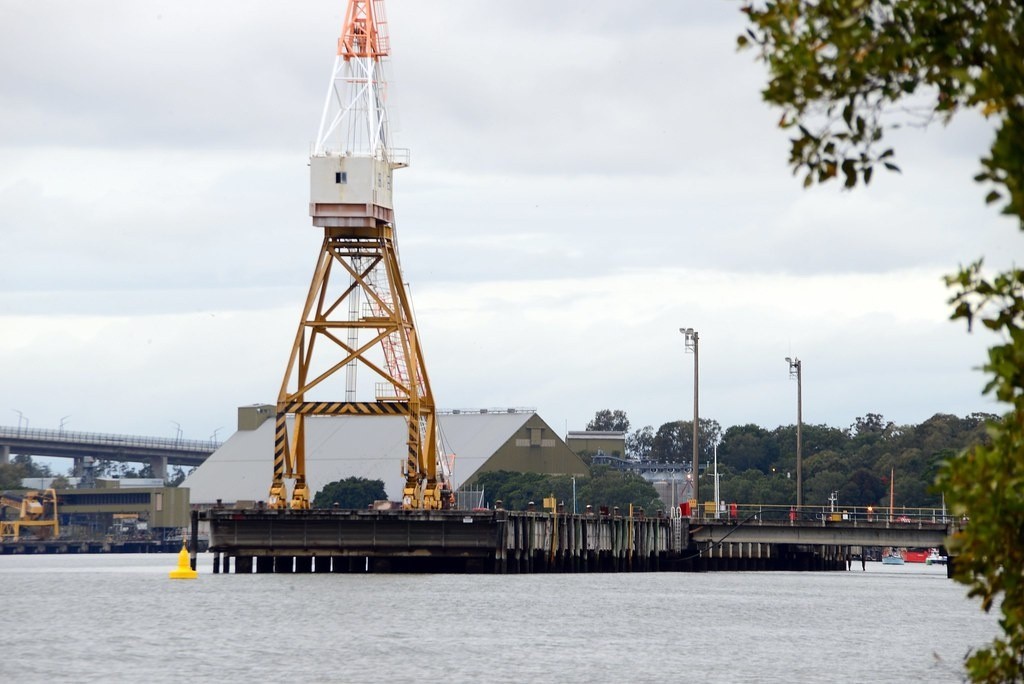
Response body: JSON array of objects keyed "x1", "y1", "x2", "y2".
[
  {"x1": 785, "y1": 355, "x2": 807, "y2": 520},
  {"x1": 679, "y1": 327, "x2": 699, "y2": 512}
]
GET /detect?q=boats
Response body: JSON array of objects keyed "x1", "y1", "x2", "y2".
[
  {"x1": 927, "y1": 548, "x2": 949, "y2": 565},
  {"x1": 882, "y1": 553, "x2": 904, "y2": 565},
  {"x1": 900, "y1": 548, "x2": 929, "y2": 563}
]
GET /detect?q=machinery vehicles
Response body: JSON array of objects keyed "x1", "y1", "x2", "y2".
[{"x1": 0, "y1": 486, "x2": 59, "y2": 539}]
